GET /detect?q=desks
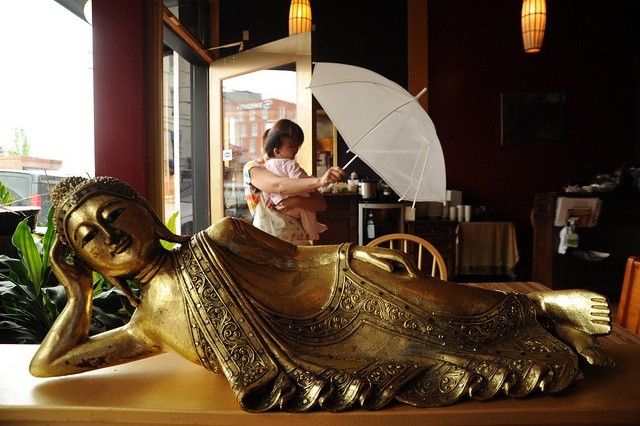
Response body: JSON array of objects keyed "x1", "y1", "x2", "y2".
[{"x1": 458, "y1": 281, "x2": 640, "y2": 347}]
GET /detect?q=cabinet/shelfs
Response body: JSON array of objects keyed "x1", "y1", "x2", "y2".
[
  {"x1": 313, "y1": 193, "x2": 362, "y2": 244},
  {"x1": 530, "y1": 183, "x2": 625, "y2": 291},
  {"x1": 407, "y1": 219, "x2": 453, "y2": 279},
  {"x1": 456, "y1": 222, "x2": 519, "y2": 282}
]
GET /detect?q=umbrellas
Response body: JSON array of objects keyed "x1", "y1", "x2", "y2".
[{"x1": 306, "y1": 61, "x2": 447, "y2": 202}]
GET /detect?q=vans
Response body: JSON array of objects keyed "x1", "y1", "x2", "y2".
[{"x1": 0, "y1": 169, "x2": 89, "y2": 235}]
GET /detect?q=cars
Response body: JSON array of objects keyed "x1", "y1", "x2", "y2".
[{"x1": 180, "y1": 183, "x2": 250, "y2": 236}]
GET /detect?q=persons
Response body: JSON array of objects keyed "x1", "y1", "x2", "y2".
[
  {"x1": 29, "y1": 176, "x2": 615, "y2": 414},
  {"x1": 243, "y1": 120, "x2": 343, "y2": 246},
  {"x1": 264, "y1": 134, "x2": 327, "y2": 240}
]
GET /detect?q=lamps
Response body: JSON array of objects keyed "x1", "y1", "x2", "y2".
[
  {"x1": 289, "y1": 0, "x2": 312, "y2": 35},
  {"x1": 520, "y1": 0, "x2": 547, "y2": 53}
]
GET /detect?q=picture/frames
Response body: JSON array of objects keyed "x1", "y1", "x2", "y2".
[{"x1": 500, "y1": 91, "x2": 561, "y2": 146}]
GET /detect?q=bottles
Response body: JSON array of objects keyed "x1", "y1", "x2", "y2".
[{"x1": 366, "y1": 211, "x2": 375, "y2": 238}]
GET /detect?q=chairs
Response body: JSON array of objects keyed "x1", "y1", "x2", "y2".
[
  {"x1": 365, "y1": 233, "x2": 448, "y2": 281},
  {"x1": 617, "y1": 256, "x2": 640, "y2": 336}
]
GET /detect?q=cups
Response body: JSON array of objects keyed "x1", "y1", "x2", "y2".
[{"x1": 348, "y1": 180, "x2": 358, "y2": 193}]
